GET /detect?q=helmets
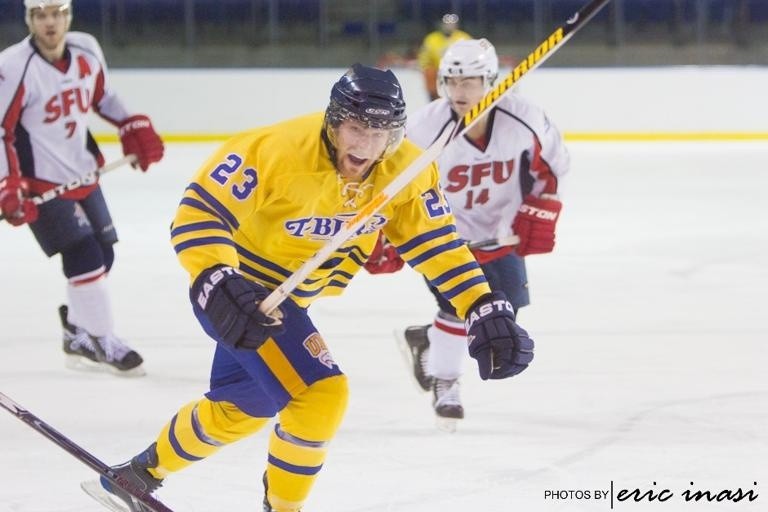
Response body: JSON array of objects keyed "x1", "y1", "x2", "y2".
[
  {"x1": 435, "y1": 37, "x2": 498, "y2": 105},
  {"x1": 23, "y1": 0, "x2": 71, "y2": 30},
  {"x1": 323, "y1": 62, "x2": 407, "y2": 160}
]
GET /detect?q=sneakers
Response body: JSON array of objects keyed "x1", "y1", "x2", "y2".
[
  {"x1": 432, "y1": 379, "x2": 464, "y2": 419},
  {"x1": 92, "y1": 334, "x2": 143, "y2": 371},
  {"x1": 62, "y1": 329, "x2": 100, "y2": 362},
  {"x1": 404, "y1": 323, "x2": 434, "y2": 391},
  {"x1": 100, "y1": 456, "x2": 164, "y2": 512}
]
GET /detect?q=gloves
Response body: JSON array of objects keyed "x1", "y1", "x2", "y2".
[
  {"x1": 511, "y1": 195, "x2": 562, "y2": 257},
  {"x1": 120, "y1": 115, "x2": 164, "y2": 172},
  {"x1": 189, "y1": 263, "x2": 288, "y2": 350},
  {"x1": 362, "y1": 229, "x2": 405, "y2": 274},
  {"x1": 0, "y1": 176, "x2": 38, "y2": 226},
  {"x1": 463, "y1": 292, "x2": 533, "y2": 381}
]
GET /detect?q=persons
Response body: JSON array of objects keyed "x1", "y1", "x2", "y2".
[
  {"x1": 0, "y1": 0, "x2": 162, "y2": 372},
  {"x1": 417, "y1": 7, "x2": 479, "y2": 104},
  {"x1": 98, "y1": 64, "x2": 537, "y2": 512},
  {"x1": 403, "y1": 38, "x2": 571, "y2": 419}
]
